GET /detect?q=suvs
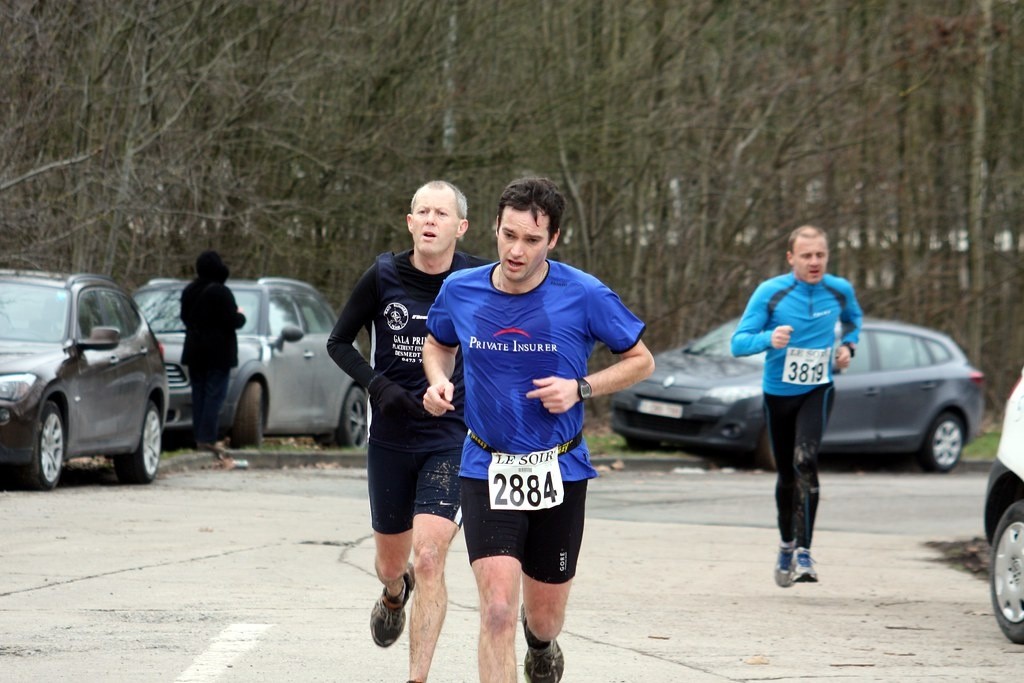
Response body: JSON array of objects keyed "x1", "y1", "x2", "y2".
[
  {"x1": 1, "y1": 267, "x2": 170, "y2": 493},
  {"x1": 126, "y1": 276, "x2": 372, "y2": 449}
]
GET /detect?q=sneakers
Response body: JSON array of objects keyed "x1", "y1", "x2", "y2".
[
  {"x1": 521, "y1": 603, "x2": 564, "y2": 683},
  {"x1": 774, "y1": 538, "x2": 796, "y2": 587},
  {"x1": 790, "y1": 547, "x2": 818, "y2": 582},
  {"x1": 369, "y1": 563, "x2": 415, "y2": 647}
]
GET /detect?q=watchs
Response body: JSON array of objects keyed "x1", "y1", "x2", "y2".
[
  {"x1": 842, "y1": 342, "x2": 855, "y2": 358},
  {"x1": 575, "y1": 377, "x2": 592, "y2": 403}
]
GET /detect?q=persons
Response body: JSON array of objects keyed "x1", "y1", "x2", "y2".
[
  {"x1": 421, "y1": 176, "x2": 655, "y2": 683},
  {"x1": 731, "y1": 225, "x2": 863, "y2": 587},
  {"x1": 178, "y1": 250, "x2": 246, "y2": 454},
  {"x1": 328, "y1": 181, "x2": 496, "y2": 681}
]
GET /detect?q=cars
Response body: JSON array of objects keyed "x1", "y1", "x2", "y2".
[
  {"x1": 610, "y1": 315, "x2": 985, "y2": 475},
  {"x1": 982, "y1": 363, "x2": 1024, "y2": 646}
]
